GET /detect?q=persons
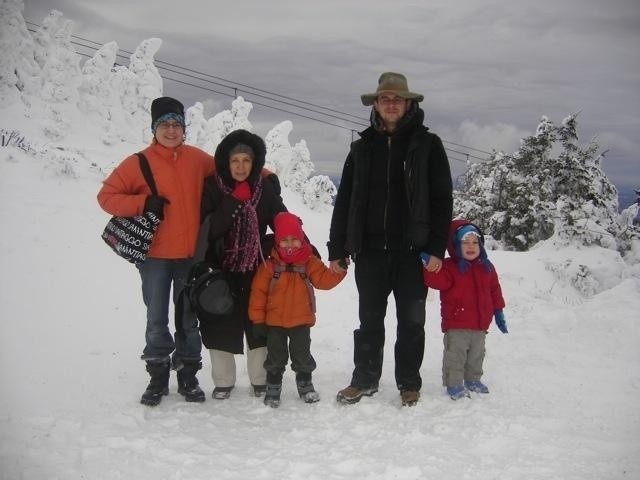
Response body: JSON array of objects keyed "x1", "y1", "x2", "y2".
[
  {"x1": 418, "y1": 219, "x2": 510, "y2": 402},
  {"x1": 188, "y1": 127, "x2": 321, "y2": 403},
  {"x1": 326, "y1": 70, "x2": 454, "y2": 410},
  {"x1": 95, "y1": 96, "x2": 281, "y2": 408},
  {"x1": 246, "y1": 211, "x2": 351, "y2": 409}
]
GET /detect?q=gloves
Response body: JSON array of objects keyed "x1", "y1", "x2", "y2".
[
  {"x1": 495, "y1": 311, "x2": 508, "y2": 333},
  {"x1": 420, "y1": 252, "x2": 429, "y2": 266},
  {"x1": 145, "y1": 195, "x2": 170, "y2": 220},
  {"x1": 252, "y1": 323, "x2": 268, "y2": 338},
  {"x1": 233, "y1": 181, "x2": 251, "y2": 200}
]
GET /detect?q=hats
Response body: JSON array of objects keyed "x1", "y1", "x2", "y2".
[
  {"x1": 273, "y1": 212, "x2": 304, "y2": 246},
  {"x1": 151, "y1": 97, "x2": 185, "y2": 135},
  {"x1": 361, "y1": 73, "x2": 424, "y2": 106},
  {"x1": 229, "y1": 143, "x2": 255, "y2": 159},
  {"x1": 453, "y1": 225, "x2": 492, "y2": 272}
]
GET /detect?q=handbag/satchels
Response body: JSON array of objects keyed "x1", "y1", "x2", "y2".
[
  {"x1": 187, "y1": 262, "x2": 237, "y2": 322},
  {"x1": 102, "y1": 212, "x2": 161, "y2": 265}
]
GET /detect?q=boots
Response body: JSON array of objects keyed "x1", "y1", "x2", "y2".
[
  {"x1": 297, "y1": 373, "x2": 320, "y2": 403},
  {"x1": 264, "y1": 373, "x2": 282, "y2": 408},
  {"x1": 141, "y1": 365, "x2": 170, "y2": 406},
  {"x1": 177, "y1": 367, "x2": 205, "y2": 402}
]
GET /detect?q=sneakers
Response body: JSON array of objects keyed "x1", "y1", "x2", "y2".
[
  {"x1": 400, "y1": 389, "x2": 420, "y2": 407},
  {"x1": 337, "y1": 385, "x2": 378, "y2": 404},
  {"x1": 251, "y1": 383, "x2": 267, "y2": 397},
  {"x1": 212, "y1": 385, "x2": 234, "y2": 399},
  {"x1": 464, "y1": 381, "x2": 488, "y2": 393},
  {"x1": 447, "y1": 384, "x2": 470, "y2": 400}
]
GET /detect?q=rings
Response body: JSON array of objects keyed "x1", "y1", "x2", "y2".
[{"x1": 435, "y1": 263, "x2": 439, "y2": 268}]
